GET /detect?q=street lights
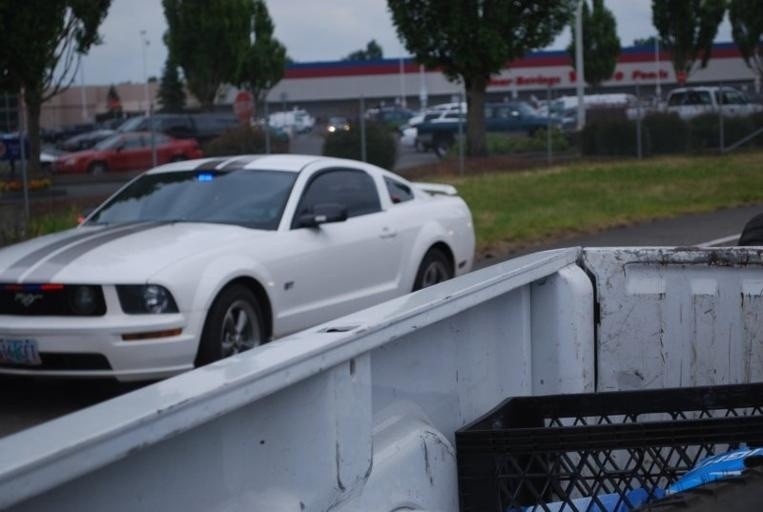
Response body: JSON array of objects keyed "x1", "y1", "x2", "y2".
[{"x1": 137, "y1": 28, "x2": 152, "y2": 116}]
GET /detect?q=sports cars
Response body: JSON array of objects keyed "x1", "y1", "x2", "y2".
[{"x1": 0, "y1": 153, "x2": 477, "y2": 398}]
